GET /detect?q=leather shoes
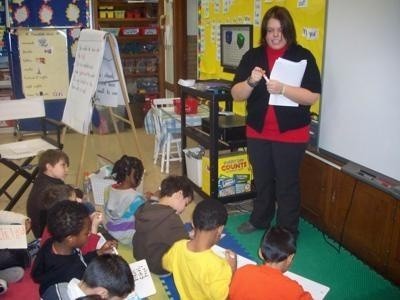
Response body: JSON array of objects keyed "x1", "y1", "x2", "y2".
[{"x1": 237, "y1": 222, "x2": 259, "y2": 234}]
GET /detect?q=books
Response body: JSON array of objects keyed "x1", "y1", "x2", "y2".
[
  {"x1": 268, "y1": 57, "x2": 308, "y2": 108},
  {"x1": 283, "y1": 270, "x2": 330, "y2": 300}
]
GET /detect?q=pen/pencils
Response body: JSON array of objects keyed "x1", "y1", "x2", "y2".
[
  {"x1": 112, "y1": 246, "x2": 119, "y2": 256},
  {"x1": 222, "y1": 251, "x2": 236, "y2": 259}
]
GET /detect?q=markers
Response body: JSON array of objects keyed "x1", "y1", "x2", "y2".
[
  {"x1": 262, "y1": 72, "x2": 270, "y2": 83},
  {"x1": 358, "y1": 171, "x2": 392, "y2": 189}
]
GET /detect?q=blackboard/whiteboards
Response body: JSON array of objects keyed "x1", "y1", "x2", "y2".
[{"x1": 314, "y1": 0, "x2": 400, "y2": 200}]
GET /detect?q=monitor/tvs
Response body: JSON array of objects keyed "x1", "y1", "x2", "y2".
[{"x1": 220, "y1": 24, "x2": 253, "y2": 73}]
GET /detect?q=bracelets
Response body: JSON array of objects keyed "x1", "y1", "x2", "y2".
[{"x1": 280, "y1": 82, "x2": 286, "y2": 95}]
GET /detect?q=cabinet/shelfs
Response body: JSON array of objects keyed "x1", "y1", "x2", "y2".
[
  {"x1": 298, "y1": 143, "x2": 399, "y2": 291},
  {"x1": 94, "y1": 1, "x2": 167, "y2": 129},
  {"x1": 178, "y1": 78, "x2": 257, "y2": 210}
]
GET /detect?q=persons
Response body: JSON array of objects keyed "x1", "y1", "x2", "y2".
[
  {"x1": 161, "y1": 197, "x2": 236, "y2": 300},
  {"x1": 133, "y1": 175, "x2": 194, "y2": 275},
  {"x1": 231, "y1": 5, "x2": 321, "y2": 247},
  {"x1": 0, "y1": 148, "x2": 161, "y2": 300},
  {"x1": 229, "y1": 224, "x2": 314, "y2": 300}
]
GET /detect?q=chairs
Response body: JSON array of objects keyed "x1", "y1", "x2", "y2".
[
  {"x1": 0, "y1": 94, "x2": 66, "y2": 212},
  {"x1": 149, "y1": 97, "x2": 209, "y2": 174}
]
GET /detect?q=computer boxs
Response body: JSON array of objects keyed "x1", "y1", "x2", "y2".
[{"x1": 202, "y1": 115, "x2": 246, "y2": 142}]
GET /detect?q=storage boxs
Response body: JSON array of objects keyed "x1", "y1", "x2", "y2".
[
  {"x1": 182, "y1": 146, "x2": 205, "y2": 188},
  {"x1": 201, "y1": 146, "x2": 253, "y2": 199}
]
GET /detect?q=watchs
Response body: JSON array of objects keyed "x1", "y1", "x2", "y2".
[{"x1": 247, "y1": 76, "x2": 257, "y2": 87}]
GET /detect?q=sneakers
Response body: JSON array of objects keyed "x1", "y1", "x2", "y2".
[
  {"x1": 27, "y1": 238, "x2": 42, "y2": 256},
  {"x1": 0, "y1": 266, "x2": 24, "y2": 284}
]
